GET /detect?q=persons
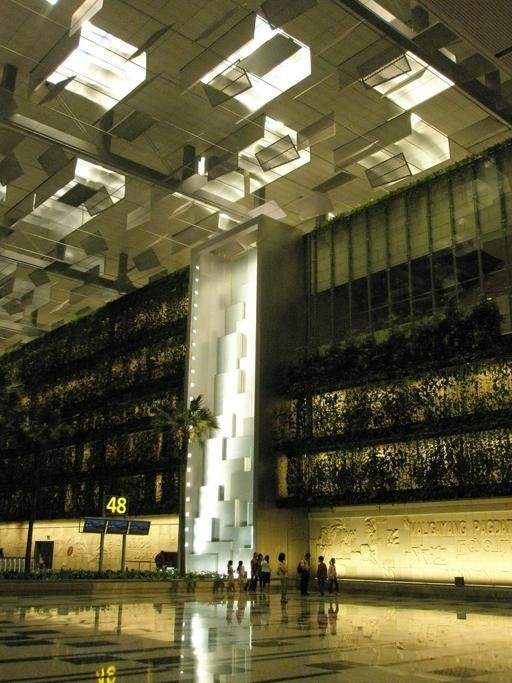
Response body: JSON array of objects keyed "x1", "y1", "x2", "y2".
[
  {"x1": 249, "y1": 593, "x2": 261, "y2": 629},
  {"x1": 327, "y1": 597, "x2": 339, "y2": 635},
  {"x1": 276, "y1": 551, "x2": 290, "y2": 603},
  {"x1": 297, "y1": 552, "x2": 312, "y2": 595},
  {"x1": 225, "y1": 558, "x2": 237, "y2": 592},
  {"x1": 236, "y1": 559, "x2": 247, "y2": 592},
  {"x1": 327, "y1": 557, "x2": 341, "y2": 596},
  {"x1": 260, "y1": 593, "x2": 272, "y2": 632},
  {"x1": 260, "y1": 554, "x2": 272, "y2": 593},
  {"x1": 235, "y1": 593, "x2": 248, "y2": 625},
  {"x1": 316, "y1": 598, "x2": 328, "y2": 639},
  {"x1": 253, "y1": 552, "x2": 264, "y2": 591},
  {"x1": 298, "y1": 598, "x2": 313, "y2": 638},
  {"x1": 316, "y1": 554, "x2": 328, "y2": 597},
  {"x1": 276, "y1": 602, "x2": 289, "y2": 635},
  {"x1": 225, "y1": 592, "x2": 236, "y2": 624},
  {"x1": 247, "y1": 551, "x2": 258, "y2": 591}
]
